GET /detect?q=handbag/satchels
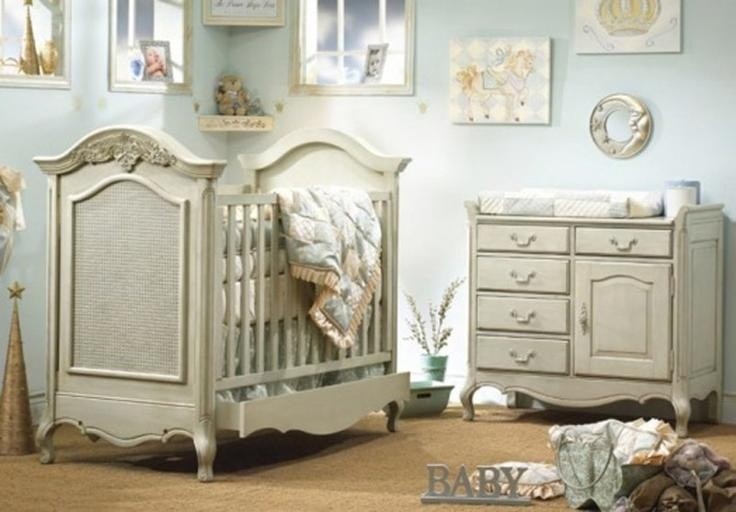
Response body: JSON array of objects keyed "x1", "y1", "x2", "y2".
[{"x1": 547, "y1": 417, "x2": 678, "y2": 512}]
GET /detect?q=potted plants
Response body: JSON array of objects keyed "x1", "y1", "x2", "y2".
[{"x1": 400, "y1": 275, "x2": 467, "y2": 383}]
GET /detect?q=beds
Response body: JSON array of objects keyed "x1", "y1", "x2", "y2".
[{"x1": 32, "y1": 126, "x2": 412, "y2": 484}]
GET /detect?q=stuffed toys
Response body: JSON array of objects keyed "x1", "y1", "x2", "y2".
[{"x1": 213, "y1": 74, "x2": 249, "y2": 116}]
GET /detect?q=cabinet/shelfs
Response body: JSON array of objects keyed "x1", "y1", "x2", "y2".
[{"x1": 458, "y1": 199, "x2": 725, "y2": 439}]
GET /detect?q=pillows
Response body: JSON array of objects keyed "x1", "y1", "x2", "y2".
[{"x1": 468, "y1": 461, "x2": 565, "y2": 500}]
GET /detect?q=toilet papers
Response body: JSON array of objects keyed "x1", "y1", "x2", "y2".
[{"x1": 662, "y1": 186, "x2": 697, "y2": 218}]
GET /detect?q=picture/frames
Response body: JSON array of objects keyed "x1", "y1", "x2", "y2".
[
  {"x1": 138, "y1": 38, "x2": 171, "y2": 82},
  {"x1": 360, "y1": 43, "x2": 387, "y2": 84},
  {"x1": 200, "y1": 0, "x2": 286, "y2": 28}
]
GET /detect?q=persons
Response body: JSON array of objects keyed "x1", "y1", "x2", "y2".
[
  {"x1": 143, "y1": 46, "x2": 166, "y2": 81},
  {"x1": 365, "y1": 49, "x2": 383, "y2": 76}
]
